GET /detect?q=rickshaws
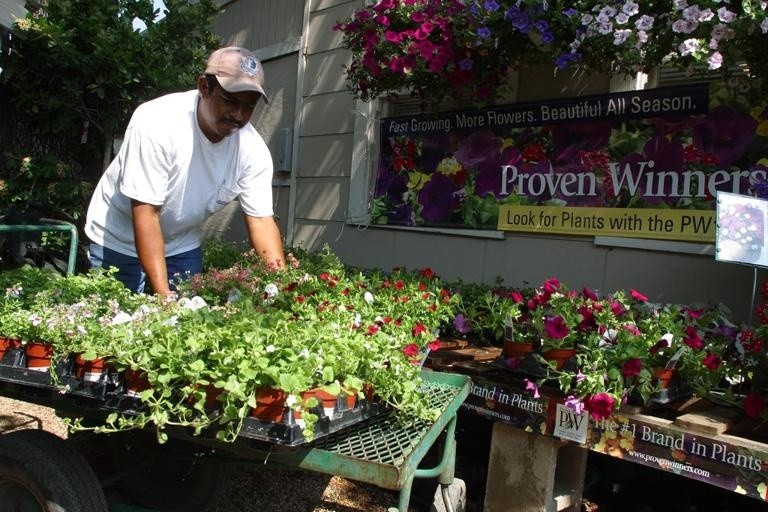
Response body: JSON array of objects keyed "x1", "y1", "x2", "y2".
[{"x1": 1, "y1": 216, "x2": 473, "y2": 511}]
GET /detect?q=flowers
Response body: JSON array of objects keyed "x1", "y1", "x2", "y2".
[{"x1": 0, "y1": 241, "x2": 768, "y2": 445}]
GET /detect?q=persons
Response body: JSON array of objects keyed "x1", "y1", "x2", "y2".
[{"x1": 80, "y1": 44, "x2": 292, "y2": 310}]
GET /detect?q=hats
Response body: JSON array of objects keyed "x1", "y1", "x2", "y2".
[{"x1": 204, "y1": 46, "x2": 269, "y2": 105}]
